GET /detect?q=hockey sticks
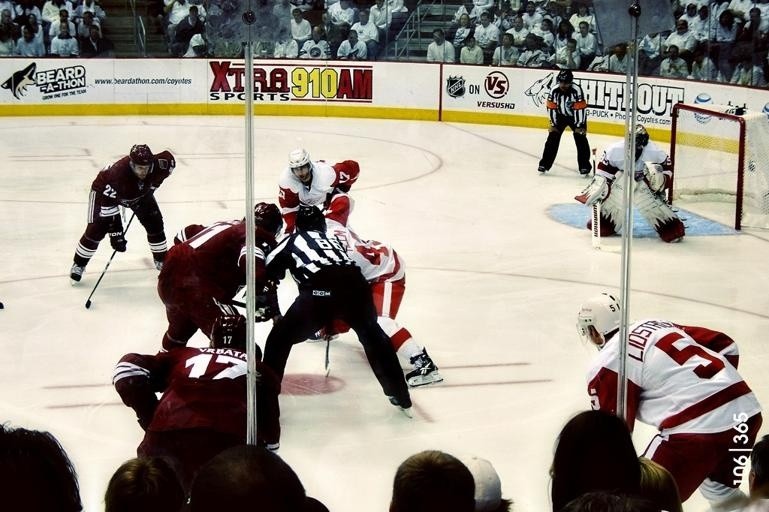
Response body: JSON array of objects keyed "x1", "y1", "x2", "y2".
[
  {"x1": 324, "y1": 323, "x2": 330, "y2": 378},
  {"x1": 86, "y1": 199, "x2": 146, "y2": 308},
  {"x1": 593, "y1": 148, "x2": 622, "y2": 251}
]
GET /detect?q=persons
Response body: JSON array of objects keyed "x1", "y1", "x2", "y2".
[
  {"x1": 633, "y1": 455, "x2": 684, "y2": 512},
  {"x1": 586, "y1": 123, "x2": 686, "y2": 243},
  {"x1": 1, "y1": 422, "x2": 84, "y2": 512},
  {"x1": 277, "y1": 148, "x2": 362, "y2": 235},
  {"x1": 637, "y1": 1, "x2": 769, "y2": 87},
  {"x1": 575, "y1": 289, "x2": 763, "y2": 511},
  {"x1": 67, "y1": 143, "x2": 176, "y2": 286},
  {"x1": 303, "y1": 194, "x2": 445, "y2": 388},
  {"x1": 159, "y1": 0, "x2": 409, "y2": 61},
  {"x1": 718, "y1": 434, "x2": 769, "y2": 512},
  {"x1": 536, "y1": 68, "x2": 592, "y2": 175},
  {"x1": 112, "y1": 314, "x2": 282, "y2": 479},
  {"x1": 427, "y1": 1, "x2": 637, "y2": 74},
  {"x1": 154, "y1": 202, "x2": 284, "y2": 374},
  {"x1": 256, "y1": 204, "x2": 414, "y2": 451},
  {"x1": 103, "y1": 455, "x2": 188, "y2": 512},
  {"x1": 1, "y1": 0, "x2": 115, "y2": 58},
  {"x1": 186, "y1": 443, "x2": 308, "y2": 512},
  {"x1": 451, "y1": 453, "x2": 515, "y2": 511},
  {"x1": 386, "y1": 450, "x2": 478, "y2": 511},
  {"x1": 548, "y1": 409, "x2": 644, "y2": 512}
]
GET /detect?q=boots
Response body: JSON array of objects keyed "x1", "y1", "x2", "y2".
[
  {"x1": 405, "y1": 347, "x2": 438, "y2": 380},
  {"x1": 307, "y1": 332, "x2": 340, "y2": 343}
]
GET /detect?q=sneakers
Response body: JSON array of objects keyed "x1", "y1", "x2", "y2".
[
  {"x1": 580, "y1": 164, "x2": 591, "y2": 175},
  {"x1": 387, "y1": 394, "x2": 412, "y2": 408},
  {"x1": 538, "y1": 164, "x2": 546, "y2": 172},
  {"x1": 71, "y1": 261, "x2": 83, "y2": 280}
]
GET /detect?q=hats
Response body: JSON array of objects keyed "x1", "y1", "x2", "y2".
[{"x1": 461, "y1": 457, "x2": 501, "y2": 503}]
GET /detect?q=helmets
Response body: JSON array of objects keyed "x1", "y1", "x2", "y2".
[
  {"x1": 636, "y1": 124, "x2": 648, "y2": 148},
  {"x1": 129, "y1": 143, "x2": 153, "y2": 166},
  {"x1": 287, "y1": 148, "x2": 312, "y2": 176},
  {"x1": 295, "y1": 205, "x2": 325, "y2": 231},
  {"x1": 575, "y1": 289, "x2": 622, "y2": 349},
  {"x1": 555, "y1": 68, "x2": 574, "y2": 82},
  {"x1": 210, "y1": 315, "x2": 247, "y2": 353},
  {"x1": 255, "y1": 202, "x2": 283, "y2": 235}
]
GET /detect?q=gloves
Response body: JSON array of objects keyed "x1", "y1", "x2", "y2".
[
  {"x1": 549, "y1": 124, "x2": 558, "y2": 134},
  {"x1": 110, "y1": 232, "x2": 126, "y2": 253},
  {"x1": 575, "y1": 127, "x2": 586, "y2": 136}
]
